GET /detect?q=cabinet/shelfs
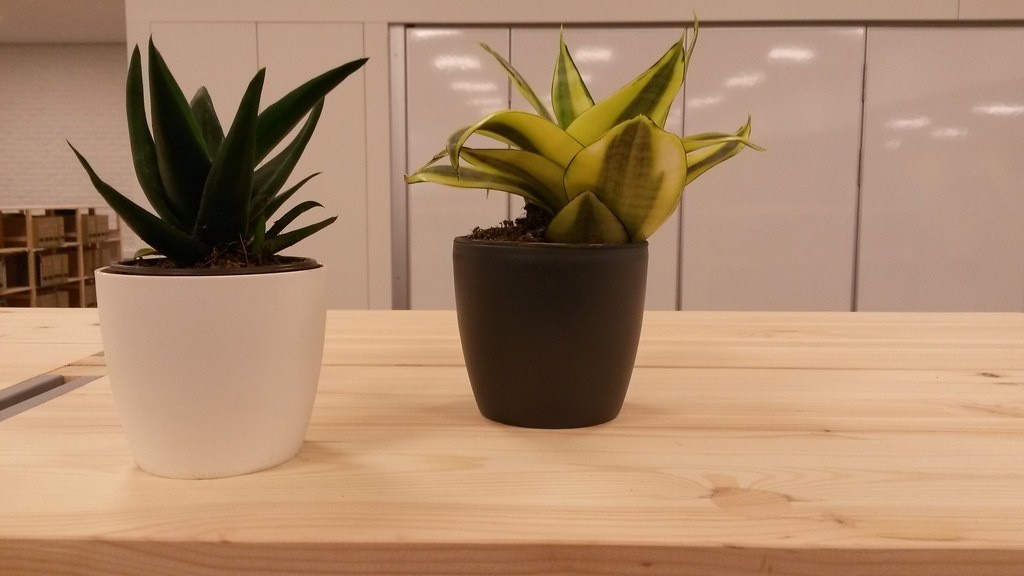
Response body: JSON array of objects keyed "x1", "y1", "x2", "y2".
[
  {"x1": 0, "y1": 206, "x2": 122, "y2": 308},
  {"x1": 126, "y1": 0, "x2": 1024, "y2": 310}
]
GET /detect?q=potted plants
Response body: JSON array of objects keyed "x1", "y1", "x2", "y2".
[
  {"x1": 67, "y1": 37, "x2": 369, "y2": 479},
  {"x1": 401, "y1": 8, "x2": 766, "y2": 429}
]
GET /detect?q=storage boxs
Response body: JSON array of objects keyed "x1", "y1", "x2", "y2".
[
  {"x1": 8, "y1": 290, "x2": 69, "y2": 307},
  {"x1": 82, "y1": 214, "x2": 109, "y2": 237},
  {"x1": 67, "y1": 248, "x2": 111, "y2": 279},
  {"x1": 11, "y1": 252, "x2": 68, "y2": 289},
  {"x1": 63, "y1": 284, "x2": 98, "y2": 307},
  {"x1": 2, "y1": 214, "x2": 65, "y2": 249}
]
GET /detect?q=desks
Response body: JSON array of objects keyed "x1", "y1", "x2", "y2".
[{"x1": 1, "y1": 305, "x2": 1024, "y2": 576}]
